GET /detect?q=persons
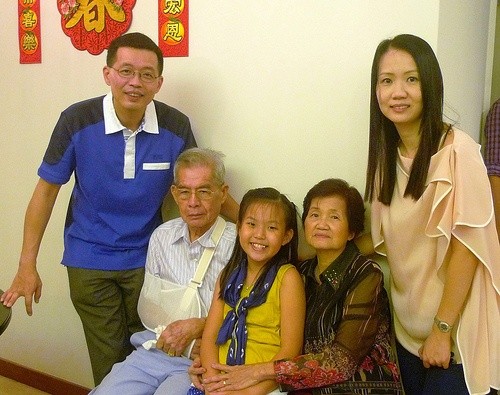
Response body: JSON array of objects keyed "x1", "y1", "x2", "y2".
[
  {"x1": 0, "y1": 32, "x2": 240, "y2": 387},
  {"x1": 87, "y1": 148, "x2": 238, "y2": 395},
  {"x1": 297, "y1": 34, "x2": 500, "y2": 395},
  {"x1": 201, "y1": 188, "x2": 306, "y2": 395},
  {"x1": 188, "y1": 178, "x2": 405, "y2": 395},
  {"x1": 484, "y1": 98, "x2": 500, "y2": 239}
]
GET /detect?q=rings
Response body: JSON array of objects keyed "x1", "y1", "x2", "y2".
[
  {"x1": 167, "y1": 351, "x2": 175, "y2": 357},
  {"x1": 223, "y1": 380, "x2": 226, "y2": 386}
]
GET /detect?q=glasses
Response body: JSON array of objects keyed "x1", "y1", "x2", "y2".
[
  {"x1": 176, "y1": 182, "x2": 224, "y2": 199},
  {"x1": 108, "y1": 66, "x2": 160, "y2": 83}
]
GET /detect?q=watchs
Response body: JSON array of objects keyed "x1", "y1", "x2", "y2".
[{"x1": 434, "y1": 315, "x2": 453, "y2": 333}]
